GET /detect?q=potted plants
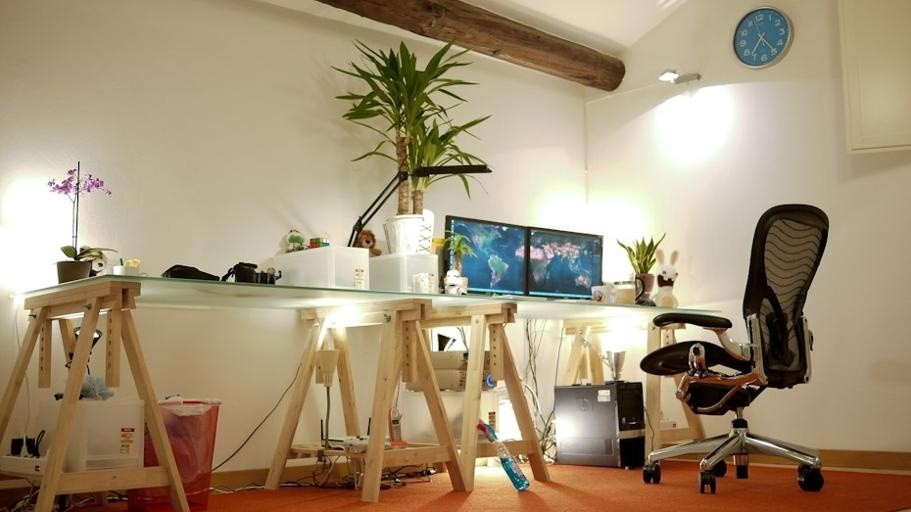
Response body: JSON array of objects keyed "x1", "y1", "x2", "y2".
[
  {"x1": 433, "y1": 228, "x2": 477, "y2": 294},
  {"x1": 330, "y1": 34, "x2": 490, "y2": 252},
  {"x1": 616, "y1": 233, "x2": 667, "y2": 306}
]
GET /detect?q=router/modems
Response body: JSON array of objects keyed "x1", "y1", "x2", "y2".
[{"x1": 321, "y1": 417, "x2": 371, "y2": 450}]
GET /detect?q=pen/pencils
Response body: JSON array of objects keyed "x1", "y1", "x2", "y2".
[{"x1": 120, "y1": 258, "x2": 123, "y2": 265}]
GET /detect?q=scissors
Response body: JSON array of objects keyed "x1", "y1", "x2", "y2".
[{"x1": 124, "y1": 259, "x2": 141, "y2": 268}]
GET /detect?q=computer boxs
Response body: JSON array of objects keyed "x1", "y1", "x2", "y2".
[{"x1": 554, "y1": 381, "x2": 646, "y2": 469}]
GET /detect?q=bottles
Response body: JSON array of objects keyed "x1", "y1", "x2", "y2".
[{"x1": 355, "y1": 268, "x2": 364, "y2": 290}]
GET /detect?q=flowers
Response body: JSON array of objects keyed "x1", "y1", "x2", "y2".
[{"x1": 48, "y1": 160, "x2": 119, "y2": 261}]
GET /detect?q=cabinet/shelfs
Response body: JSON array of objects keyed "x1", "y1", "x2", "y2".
[{"x1": 837, "y1": 0, "x2": 911, "y2": 155}]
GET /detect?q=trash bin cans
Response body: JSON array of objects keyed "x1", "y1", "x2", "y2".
[{"x1": 127, "y1": 393, "x2": 222, "y2": 512}]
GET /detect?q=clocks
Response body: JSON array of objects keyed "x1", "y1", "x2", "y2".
[{"x1": 731, "y1": 6, "x2": 791, "y2": 69}]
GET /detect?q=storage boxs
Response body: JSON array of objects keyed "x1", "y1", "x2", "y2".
[
  {"x1": 273, "y1": 244, "x2": 371, "y2": 291},
  {"x1": 30, "y1": 396, "x2": 145, "y2": 472},
  {"x1": 369, "y1": 253, "x2": 440, "y2": 295}
]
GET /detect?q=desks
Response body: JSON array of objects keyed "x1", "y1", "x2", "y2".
[{"x1": 0, "y1": 275, "x2": 721, "y2": 512}]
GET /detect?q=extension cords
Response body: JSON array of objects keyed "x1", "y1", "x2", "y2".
[{"x1": 1, "y1": 453, "x2": 68, "y2": 477}]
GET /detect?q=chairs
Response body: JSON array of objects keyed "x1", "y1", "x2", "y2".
[{"x1": 641, "y1": 203, "x2": 830, "y2": 494}]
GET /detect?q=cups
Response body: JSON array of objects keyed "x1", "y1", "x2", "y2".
[
  {"x1": 607, "y1": 351, "x2": 626, "y2": 381},
  {"x1": 410, "y1": 273, "x2": 434, "y2": 295},
  {"x1": 113, "y1": 266, "x2": 137, "y2": 276},
  {"x1": 591, "y1": 281, "x2": 636, "y2": 304}
]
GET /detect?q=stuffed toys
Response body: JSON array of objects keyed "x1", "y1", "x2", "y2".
[
  {"x1": 655, "y1": 248, "x2": 680, "y2": 307},
  {"x1": 355, "y1": 230, "x2": 383, "y2": 258},
  {"x1": 287, "y1": 229, "x2": 306, "y2": 252},
  {"x1": 262, "y1": 267, "x2": 282, "y2": 283}
]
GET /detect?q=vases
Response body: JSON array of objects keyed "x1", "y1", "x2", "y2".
[{"x1": 57, "y1": 262, "x2": 91, "y2": 284}]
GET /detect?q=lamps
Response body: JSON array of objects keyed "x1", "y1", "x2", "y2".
[{"x1": 658, "y1": 69, "x2": 701, "y2": 84}]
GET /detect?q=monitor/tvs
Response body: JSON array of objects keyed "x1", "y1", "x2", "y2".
[
  {"x1": 527, "y1": 226, "x2": 604, "y2": 301},
  {"x1": 445, "y1": 215, "x2": 529, "y2": 296}
]
